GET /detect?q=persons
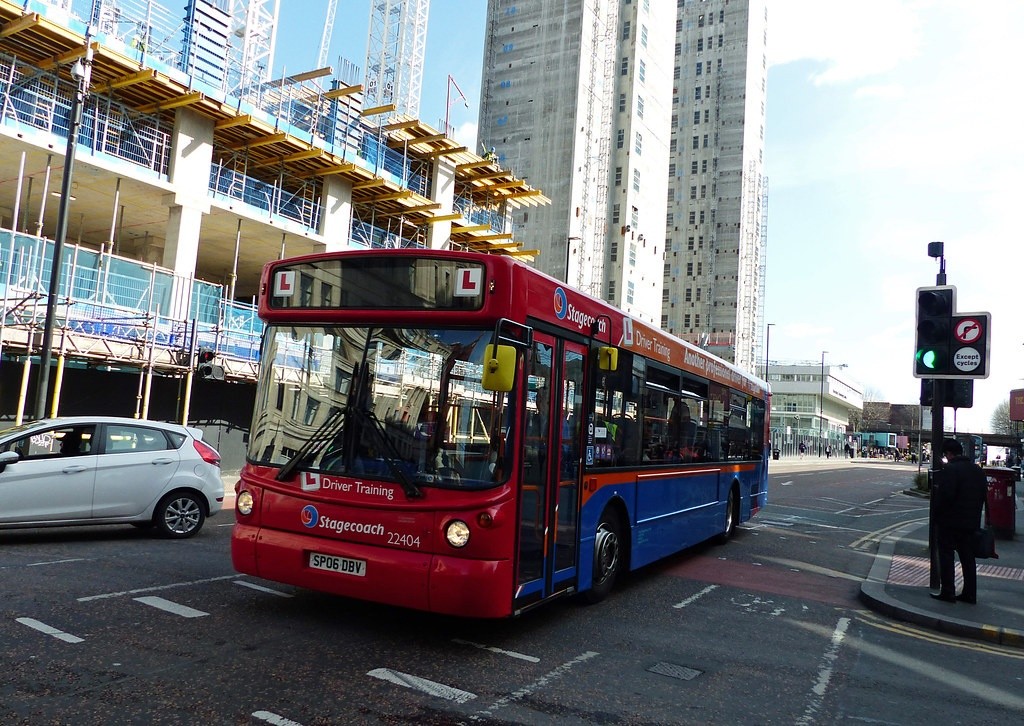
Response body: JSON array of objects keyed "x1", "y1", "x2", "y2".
[
  {"x1": 799, "y1": 441, "x2": 806, "y2": 459},
  {"x1": 911, "y1": 451, "x2": 917, "y2": 463},
  {"x1": 930, "y1": 439, "x2": 987, "y2": 606},
  {"x1": 56, "y1": 431, "x2": 82, "y2": 454},
  {"x1": 845, "y1": 443, "x2": 850, "y2": 454},
  {"x1": 825, "y1": 444, "x2": 831, "y2": 458},
  {"x1": 768, "y1": 440, "x2": 772, "y2": 458},
  {"x1": 1005, "y1": 455, "x2": 1021, "y2": 468},
  {"x1": 483, "y1": 147, "x2": 496, "y2": 162},
  {"x1": 527, "y1": 387, "x2": 569, "y2": 478}
]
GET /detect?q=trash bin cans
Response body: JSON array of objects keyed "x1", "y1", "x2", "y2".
[{"x1": 773, "y1": 449, "x2": 781, "y2": 461}]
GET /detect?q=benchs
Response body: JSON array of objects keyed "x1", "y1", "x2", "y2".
[{"x1": 135, "y1": 435, "x2": 180, "y2": 450}]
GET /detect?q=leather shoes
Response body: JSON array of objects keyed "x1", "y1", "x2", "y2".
[
  {"x1": 957, "y1": 593, "x2": 977, "y2": 604},
  {"x1": 929, "y1": 590, "x2": 955, "y2": 603}
]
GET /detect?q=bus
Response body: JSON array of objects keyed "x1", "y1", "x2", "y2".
[
  {"x1": 230, "y1": 247, "x2": 772, "y2": 617},
  {"x1": 946, "y1": 433, "x2": 987, "y2": 467}
]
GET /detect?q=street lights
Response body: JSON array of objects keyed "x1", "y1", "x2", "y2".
[
  {"x1": 565, "y1": 237, "x2": 581, "y2": 283},
  {"x1": 766, "y1": 323, "x2": 776, "y2": 382},
  {"x1": 819, "y1": 351, "x2": 829, "y2": 457},
  {"x1": 928, "y1": 240, "x2": 945, "y2": 468}
]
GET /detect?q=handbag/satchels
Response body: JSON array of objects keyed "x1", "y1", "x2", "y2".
[{"x1": 975, "y1": 527, "x2": 999, "y2": 559}]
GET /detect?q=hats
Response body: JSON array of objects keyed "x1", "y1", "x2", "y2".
[{"x1": 56, "y1": 433, "x2": 82, "y2": 445}]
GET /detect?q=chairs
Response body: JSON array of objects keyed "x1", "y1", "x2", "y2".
[
  {"x1": 694, "y1": 430, "x2": 706, "y2": 458},
  {"x1": 88, "y1": 431, "x2": 113, "y2": 452},
  {"x1": 611, "y1": 413, "x2": 634, "y2": 432},
  {"x1": 683, "y1": 419, "x2": 697, "y2": 461}
]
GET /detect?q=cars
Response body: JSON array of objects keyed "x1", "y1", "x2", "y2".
[{"x1": 0, "y1": 417, "x2": 225, "y2": 536}]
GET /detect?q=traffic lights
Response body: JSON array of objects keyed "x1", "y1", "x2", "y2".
[
  {"x1": 198, "y1": 348, "x2": 225, "y2": 381},
  {"x1": 912, "y1": 286, "x2": 991, "y2": 379}
]
